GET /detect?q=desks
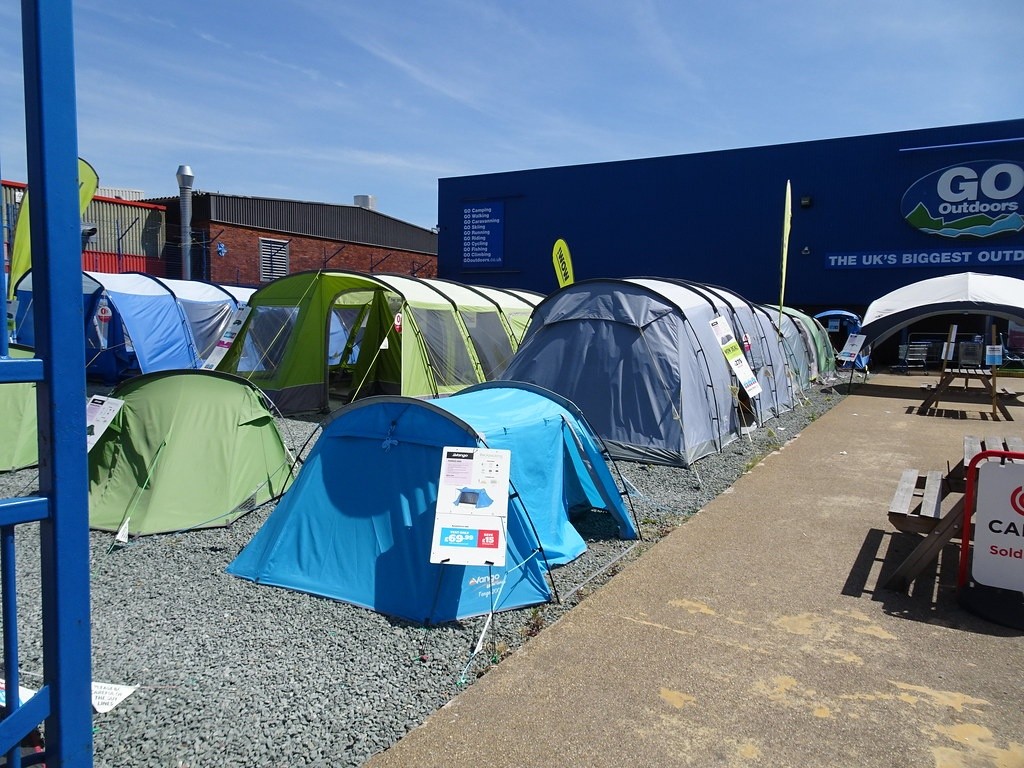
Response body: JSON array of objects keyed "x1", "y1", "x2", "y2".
[
  {"x1": 884, "y1": 435, "x2": 1024, "y2": 593},
  {"x1": 922, "y1": 369, "x2": 1013, "y2": 421}
]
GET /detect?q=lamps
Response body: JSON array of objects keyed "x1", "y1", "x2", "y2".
[{"x1": 800, "y1": 195, "x2": 811, "y2": 208}]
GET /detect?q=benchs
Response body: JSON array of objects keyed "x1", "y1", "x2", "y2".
[
  {"x1": 996, "y1": 385, "x2": 1017, "y2": 402},
  {"x1": 921, "y1": 378, "x2": 937, "y2": 397},
  {"x1": 885, "y1": 465, "x2": 947, "y2": 535}
]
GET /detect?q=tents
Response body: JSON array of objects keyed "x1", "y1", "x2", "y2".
[
  {"x1": 0, "y1": 383, "x2": 38, "y2": 472},
  {"x1": 502, "y1": 276, "x2": 837, "y2": 468},
  {"x1": 228, "y1": 382, "x2": 644, "y2": 624},
  {"x1": 215, "y1": 269, "x2": 547, "y2": 418},
  {"x1": 10, "y1": 268, "x2": 253, "y2": 380},
  {"x1": 814, "y1": 310, "x2": 872, "y2": 371},
  {"x1": 843, "y1": 272, "x2": 1024, "y2": 372},
  {"x1": 26, "y1": 369, "x2": 299, "y2": 538}
]
